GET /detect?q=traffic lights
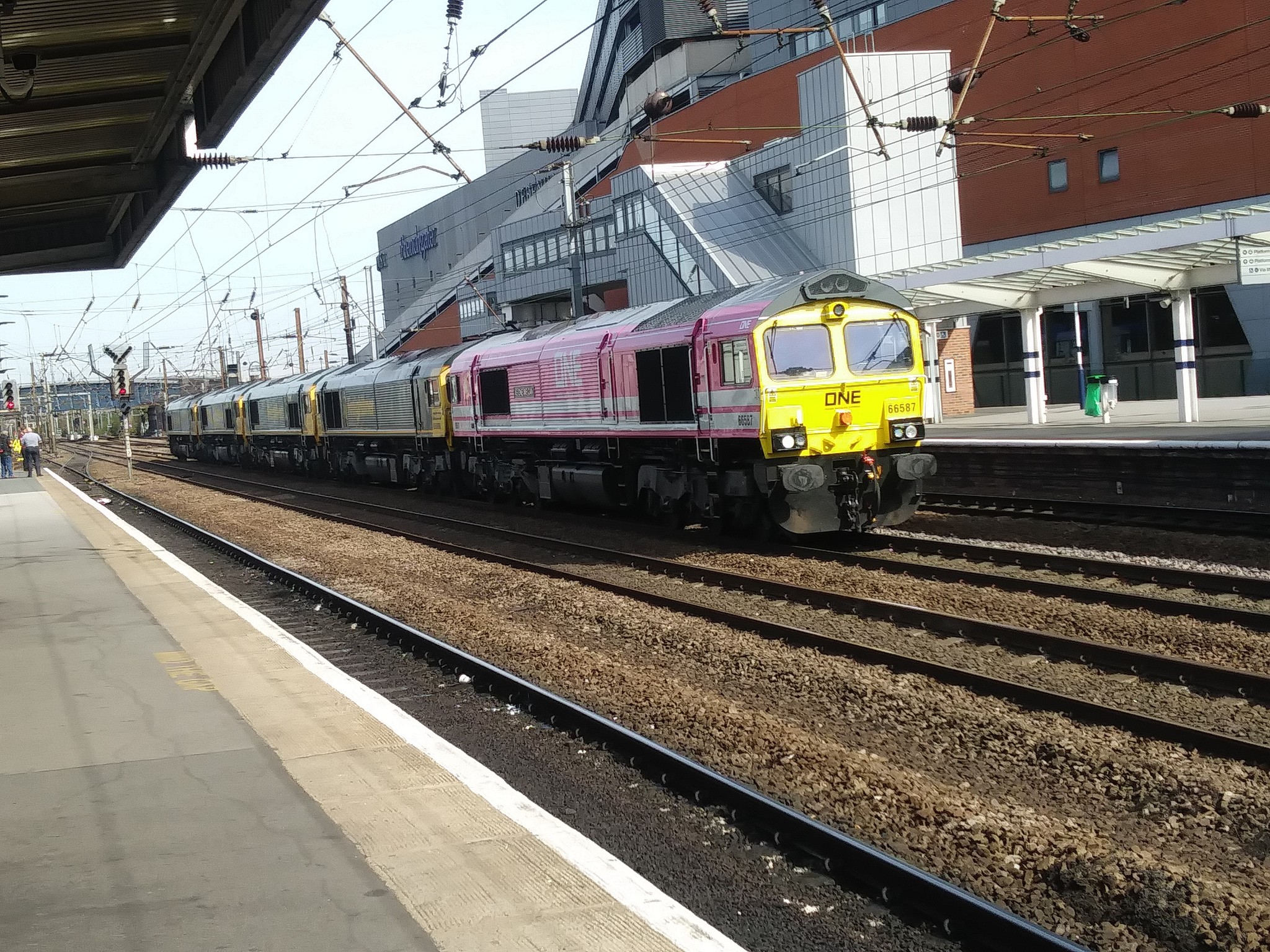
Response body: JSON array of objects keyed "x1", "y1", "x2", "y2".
[
  {"x1": 2, "y1": 380, "x2": 20, "y2": 409},
  {"x1": 112, "y1": 368, "x2": 130, "y2": 395}
]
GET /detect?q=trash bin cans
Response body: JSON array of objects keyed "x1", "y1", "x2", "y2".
[{"x1": 1085, "y1": 375, "x2": 1118, "y2": 417}]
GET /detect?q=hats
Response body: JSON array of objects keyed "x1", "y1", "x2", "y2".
[{"x1": 26, "y1": 427, "x2": 33, "y2": 432}]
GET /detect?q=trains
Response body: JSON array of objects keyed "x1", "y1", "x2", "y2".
[{"x1": 165, "y1": 268, "x2": 941, "y2": 540}]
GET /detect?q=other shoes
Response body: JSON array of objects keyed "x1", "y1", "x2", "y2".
[
  {"x1": 27, "y1": 476, "x2": 32, "y2": 478},
  {"x1": 37, "y1": 474, "x2": 44, "y2": 476}
]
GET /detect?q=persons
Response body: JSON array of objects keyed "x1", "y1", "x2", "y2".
[
  {"x1": 0, "y1": 430, "x2": 15, "y2": 478},
  {"x1": 19, "y1": 427, "x2": 43, "y2": 477}
]
